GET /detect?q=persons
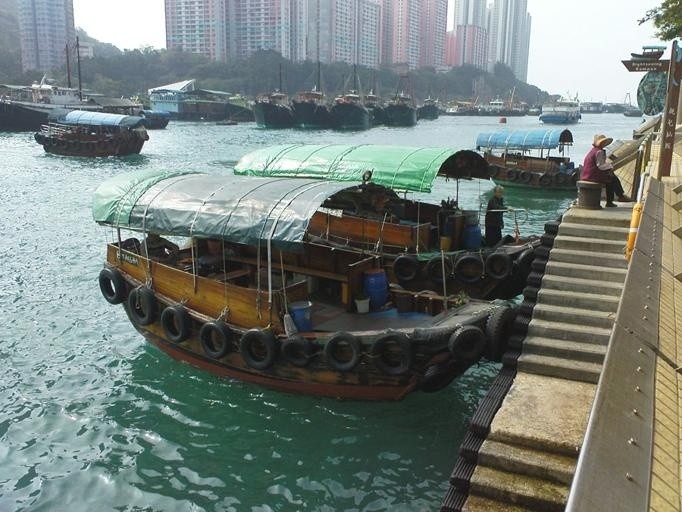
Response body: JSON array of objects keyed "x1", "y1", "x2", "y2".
[
  {"x1": 580, "y1": 134, "x2": 632, "y2": 207},
  {"x1": 196, "y1": 239, "x2": 223, "y2": 277},
  {"x1": 140, "y1": 233, "x2": 180, "y2": 266},
  {"x1": 485, "y1": 186, "x2": 514, "y2": 247}
]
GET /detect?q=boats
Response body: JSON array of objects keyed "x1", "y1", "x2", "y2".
[
  {"x1": 0, "y1": 35, "x2": 542, "y2": 131},
  {"x1": 34, "y1": 107, "x2": 150, "y2": 157},
  {"x1": 603, "y1": 102, "x2": 643, "y2": 117},
  {"x1": 538, "y1": 88, "x2": 582, "y2": 124},
  {"x1": 233, "y1": 143, "x2": 542, "y2": 300},
  {"x1": 475, "y1": 128, "x2": 583, "y2": 190},
  {"x1": 92, "y1": 168, "x2": 518, "y2": 401}
]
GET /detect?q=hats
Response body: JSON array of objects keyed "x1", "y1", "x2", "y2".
[{"x1": 594, "y1": 135, "x2": 613, "y2": 148}]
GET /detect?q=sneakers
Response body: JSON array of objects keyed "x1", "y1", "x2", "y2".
[
  {"x1": 618, "y1": 195, "x2": 633, "y2": 202},
  {"x1": 606, "y1": 203, "x2": 617, "y2": 207}
]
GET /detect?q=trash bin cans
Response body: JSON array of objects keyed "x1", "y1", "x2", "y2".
[
  {"x1": 568, "y1": 162, "x2": 574, "y2": 170},
  {"x1": 363, "y1": 266, "x2": 389, "y2": 308},
  {"x1": 287, "y1": 300, "x2": 313, "y2": 332}
]
